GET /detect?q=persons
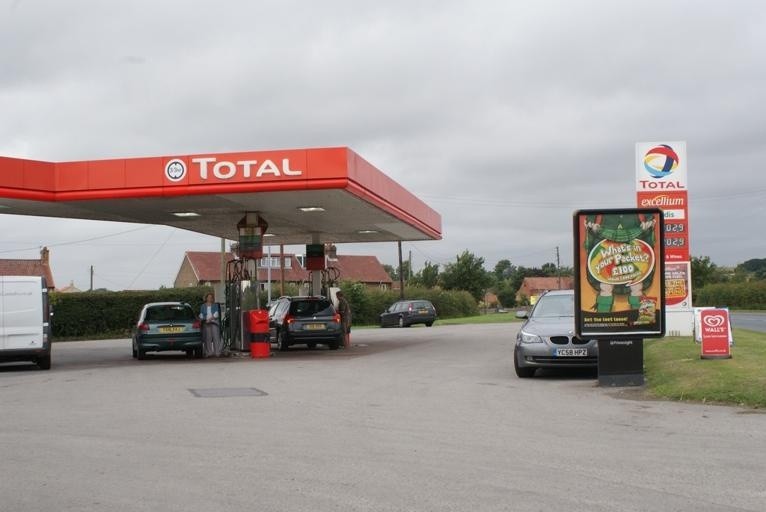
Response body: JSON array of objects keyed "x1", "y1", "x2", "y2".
[
  {"x1": 336, "y1": 290, "x2": 352, "y2": 345},
  {"x1": 198, "y1": 293, "x2": 221, "y2": 358}
]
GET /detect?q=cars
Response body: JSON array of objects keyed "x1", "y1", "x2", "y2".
[
  {"x1": 514, "y1": 289, "x2": 598, "y2": 379},
  {"x1": 380, "y1": 300, "x2": 436, "y2": 328},
  {"x1": 269, "y1": 295, "x2": 343, "y2": 351},
  {"x1": 129, "y1": 302, "x2": 202, "y2": 360}
]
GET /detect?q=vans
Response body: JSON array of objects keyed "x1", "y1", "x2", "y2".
[{"x1": 0, "y1": 276, "x2": 54, "y2": 370}]
